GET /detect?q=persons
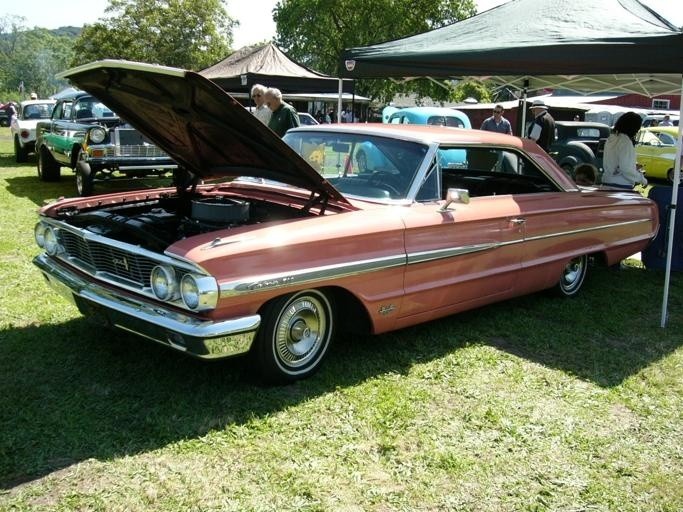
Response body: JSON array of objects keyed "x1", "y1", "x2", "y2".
[
  {"x1": 649, "y1": 115, "x2": 673, "y2": 127},
  {"x1": 30, "y1": 93, "x2": 37, "y2": 100},
  {"x1": 316, "y1": 107, "x2": 372, "y2": 124},
  {"x1": 480, "y1": 100, "x2": 583, "y2": 174},
  {"x1": 574, "y1": 112, "x2": 648, "y2": 189},
  {"x1": 251, "y1": 83, "x2": 300, "y2": 138},
  {"x1": 0, "y1": 99, "x2": 19, "y2": 126}
]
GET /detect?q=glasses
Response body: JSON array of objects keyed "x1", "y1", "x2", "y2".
[
  {"x1": 252, "y1": 94, "x2": 261, "y2": 97},
  {"x1": 494, "y1": 111, "x2": 500, "y2": 113}
]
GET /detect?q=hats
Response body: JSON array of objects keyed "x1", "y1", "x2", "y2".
[{"x1": 529, "y1": 99, "x2": 548, "y2": 109}]
